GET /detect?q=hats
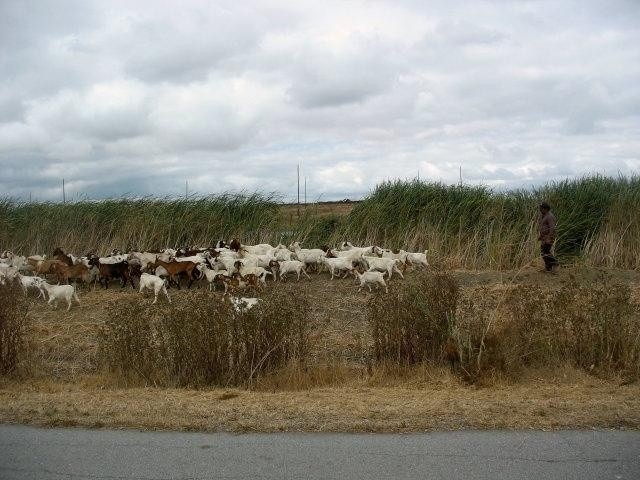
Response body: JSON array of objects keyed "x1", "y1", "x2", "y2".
[{"x1": 540, "y1": 202, "x2": 550, "y2": 208}]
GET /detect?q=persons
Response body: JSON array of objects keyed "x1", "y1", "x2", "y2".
[{"x1": 536, "y1": 201, "x2": 559, "y2": 271}]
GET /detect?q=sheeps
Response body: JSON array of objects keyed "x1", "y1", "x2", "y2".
[{"x1": 0, "y1": 236, "x2": 430, "y2": 312}]
